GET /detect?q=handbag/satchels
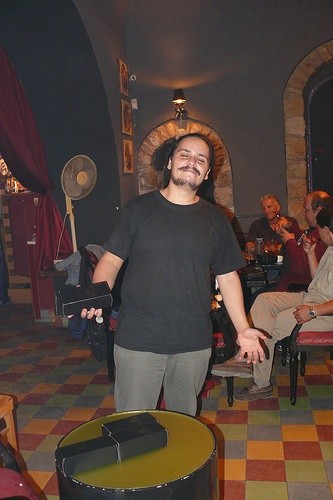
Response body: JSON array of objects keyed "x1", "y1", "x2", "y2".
[{"x1": 78, "y1": 246, "x2": 99, "y2": 286}]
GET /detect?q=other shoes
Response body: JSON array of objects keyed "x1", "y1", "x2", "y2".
[
  {"x1": 211, "y1": 357, "x2": 253, "y2": 379},
  {"x1": 234, "y1": 378, "x2": 273, "y2": 401}
]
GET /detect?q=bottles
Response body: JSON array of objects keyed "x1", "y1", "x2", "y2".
[{"x1": 256, "y1": 237, "x2": 264, "y2": 254}]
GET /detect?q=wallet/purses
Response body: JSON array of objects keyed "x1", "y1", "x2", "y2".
[{"x1": 59, "y1": 280, "x2": 114, "y2": 317}]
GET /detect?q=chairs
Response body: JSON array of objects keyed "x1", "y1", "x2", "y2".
[{"x1": 211, "y1": 280, "x2": 333, "y2": 408}]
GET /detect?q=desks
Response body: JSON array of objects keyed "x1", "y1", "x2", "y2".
[
  {"x1": 8, "y1": 189, "x2": 66, "y2": 321},
  {"x1": 55, "y1": 408, "x2": 220, "y2": 500},
  {"x1": 239, "y1": 252, "x2": 282, "y2": 309}
]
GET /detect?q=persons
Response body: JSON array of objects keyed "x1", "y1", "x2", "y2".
[
  {"x1": 245, "y1": 194, "x2": 301, "y2": 250},
  {"x1": 210, "y1": 205, "x2": 333, "y2": 400},
  {"x1": 251, "y1": 190, "x2": 332, "y2": 309},
  {"x1": 67, "y1": 132, "x2": 268, "y2": 417}
]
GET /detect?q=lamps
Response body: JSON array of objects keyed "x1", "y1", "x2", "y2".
[{"x1": 172, "y1": 88, "x2": 188, "y2": 119}]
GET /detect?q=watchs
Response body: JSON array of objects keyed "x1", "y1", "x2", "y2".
[{"x1": 309, "y1": 306, "x2": 316, "y2": 319}]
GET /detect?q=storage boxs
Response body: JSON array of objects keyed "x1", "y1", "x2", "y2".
[
  {"x1": 57, "y1": 412, "x2": 167, "y2": 475},
  {"x1": 59, "y1": 279, "x2": 114, "y2": 317}
]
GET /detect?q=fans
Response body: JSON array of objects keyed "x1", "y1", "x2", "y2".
[{"x1": 61, "y1": 154, "x2": 98, "y2": 255}]
